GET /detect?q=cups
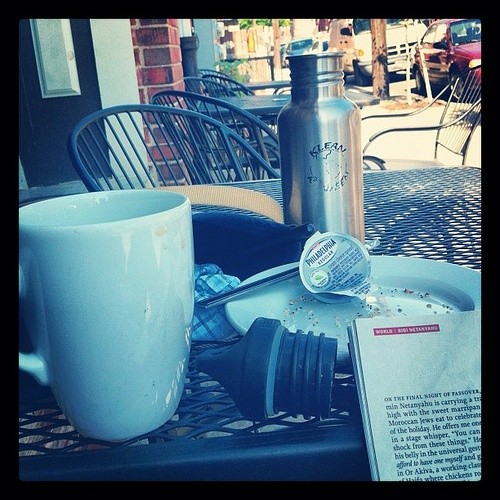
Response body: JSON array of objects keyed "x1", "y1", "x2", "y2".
[{"x1": 17, "y1": 188, "x2": 196, "y2": 441}]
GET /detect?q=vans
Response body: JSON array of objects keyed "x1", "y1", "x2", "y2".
[{"x1": 329, "y1": 19, "x2": 428, "y2": 86}]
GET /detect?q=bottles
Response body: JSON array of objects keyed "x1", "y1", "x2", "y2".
[{"x1": 277, "y1": 52, "x2": 365, "y2": 247}]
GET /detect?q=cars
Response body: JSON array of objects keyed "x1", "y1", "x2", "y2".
[
  {"x1": 409, "y1": 19, "x2": 481, "y2": 101},
  {"x1": 267, "y1": 31, "x2": 328, "y2": 67}
]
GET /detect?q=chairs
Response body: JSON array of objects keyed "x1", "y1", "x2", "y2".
[
  {"x1": 151, "y1": 87, "x2": 388, "y2": 174},
  {"x1": 181, "y1": 68, "x2": 295, "y2": 121},
  {"x1": 73, "y1": 103, "x2": 279, "y2": 195},
  {"x1": 361, "y1": 64, "x2": 481, "y2": 164}
]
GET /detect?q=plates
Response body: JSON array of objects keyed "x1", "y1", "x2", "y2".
[{"x1": 224, "y1": 253, "x2": 481, "y2": 370}]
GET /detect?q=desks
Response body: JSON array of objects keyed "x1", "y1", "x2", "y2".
[{"x1": 16, "y1": 163, "x2": 481, "y2": 479}]
